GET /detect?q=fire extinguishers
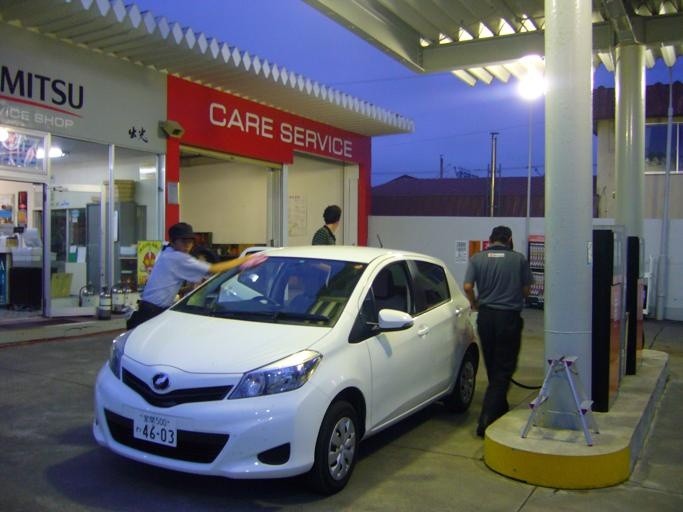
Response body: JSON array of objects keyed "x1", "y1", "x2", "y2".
[{"x1": 79, "y1": 282, "x2": 133, "y2": 320}]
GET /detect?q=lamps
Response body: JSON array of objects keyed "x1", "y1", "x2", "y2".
[{"x1": 159, "y1": 119, "x2": 185, "y2": 138}]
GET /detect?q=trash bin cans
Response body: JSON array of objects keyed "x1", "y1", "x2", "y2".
[{"x1": 9, "y1": 267, "x2": 41, "y2": 310}]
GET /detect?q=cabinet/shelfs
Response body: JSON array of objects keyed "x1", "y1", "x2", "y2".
[{"x1": 85, "y1": 200, "x2": 137, "y2": 293}]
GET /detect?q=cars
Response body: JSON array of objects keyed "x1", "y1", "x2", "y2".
[{"x1": 93, "y1": 244, "x2": 480, "y2": 495}]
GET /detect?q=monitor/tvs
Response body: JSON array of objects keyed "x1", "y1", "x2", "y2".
[{"x1": 23, "y1": 228, "x2": 41, "y2": 247}]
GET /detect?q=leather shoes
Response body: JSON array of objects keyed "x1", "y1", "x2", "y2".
[{"x1": 477, "y1": 418, "x2": 489, "y2": 435}]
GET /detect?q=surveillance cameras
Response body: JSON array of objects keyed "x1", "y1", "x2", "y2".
[{"x1": 164, "y1": 119, "x2": 184, "y2": 137}]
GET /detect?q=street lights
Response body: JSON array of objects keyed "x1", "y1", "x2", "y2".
[{"x1": 516, "y1": 71, "x2": 542, "y2": 258}]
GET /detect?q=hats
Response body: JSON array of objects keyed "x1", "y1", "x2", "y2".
[
  {"x1": 490, "y1": 226, "x2": 512, "y2": 240},
  {"x1": 169, "y1": 223, "x2": 196, "y2": 239}
]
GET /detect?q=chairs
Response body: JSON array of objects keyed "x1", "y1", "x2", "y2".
[{"x1": 287, "y1": 265, "x2": 408, "y2": 325}]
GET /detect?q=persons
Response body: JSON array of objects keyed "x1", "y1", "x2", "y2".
[
  {"x1": 464, "y1": 226, "x2": 535, "y2": 438},
  {"x1": 312, "y1": 205, "x2": 341, "y2": 245},
  {"x1": 126, "y1": 222, "x2": 254, "y2": 330}
]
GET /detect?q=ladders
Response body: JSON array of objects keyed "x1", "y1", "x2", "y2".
[{"x1": 521, "y1": 356, "x2": 600, "y2": 446}]
